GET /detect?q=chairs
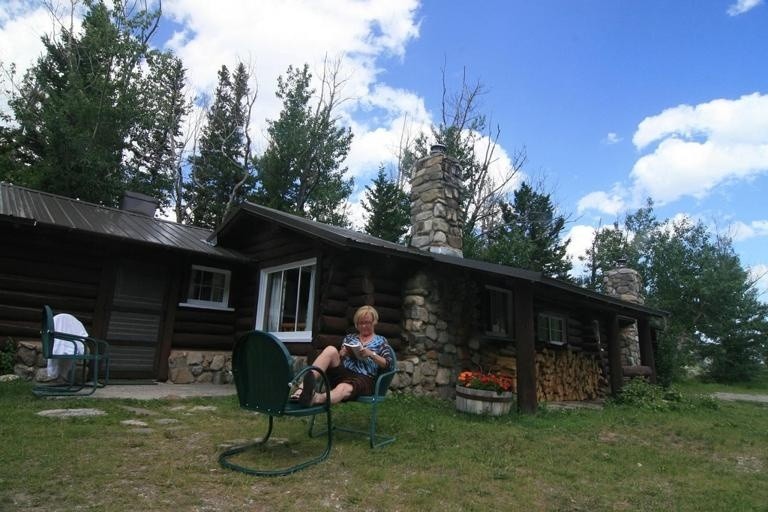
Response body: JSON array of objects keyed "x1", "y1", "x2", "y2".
[
  {"x1": 309, "y1": 342, "x2": 398, "y2": 450},
  {"x1": 217, "y1": 331, "x2": 332, "y2": 477},
  {"x1": 32, "y1": 303, "x2": 111, "y2": 396}
]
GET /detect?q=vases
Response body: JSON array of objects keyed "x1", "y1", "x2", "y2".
[{"x1": 457, "y1": 385, "x2": 513, "y2": 418}]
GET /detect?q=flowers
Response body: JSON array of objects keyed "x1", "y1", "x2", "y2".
[{"x1": 458, "y1": 368, "x2": 514, "y2": 391}]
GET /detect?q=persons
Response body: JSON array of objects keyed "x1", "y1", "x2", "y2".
[{"x1": 290, "y1": 305, "x2": 392, "y2": 408}]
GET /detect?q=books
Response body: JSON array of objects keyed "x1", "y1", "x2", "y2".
[{"x1": 343, "y1": 343, "x2": 369, "y2": 363}]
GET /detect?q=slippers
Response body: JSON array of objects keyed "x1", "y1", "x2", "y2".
[{"x1": 291, "y1": 387, "x2": 315, "y2": 400}]
